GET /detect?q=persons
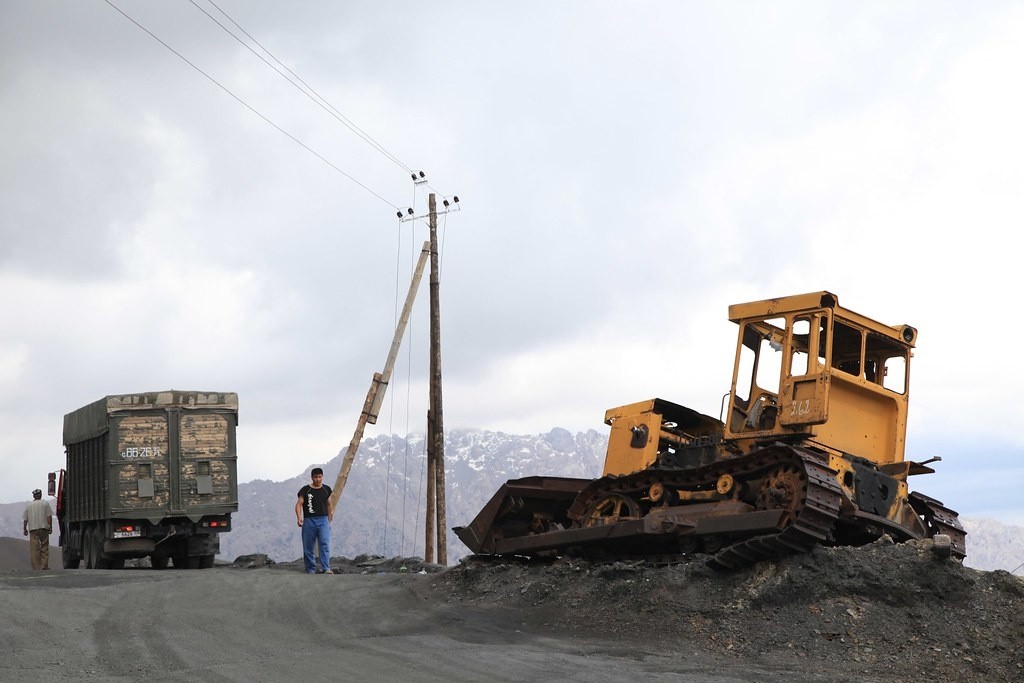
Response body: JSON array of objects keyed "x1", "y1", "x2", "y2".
[
  {"x1": 295, "y1": 468, "x2": 335, "y2": 574},
  {"x1": 22, "y1": 489, "x2": 53, "y2": 571}
]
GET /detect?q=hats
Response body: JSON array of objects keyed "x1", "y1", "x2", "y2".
[{"x1": 32, "y1": 489, "x2": 42, "y2": 497}]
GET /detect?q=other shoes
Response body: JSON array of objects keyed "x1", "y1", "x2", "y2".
[
  {"x1": 324, "y1": 570, "x2": 333, "y2": 574},
  {"x1": 308, "y1": 571, "x2": 315, "y2": 574}
]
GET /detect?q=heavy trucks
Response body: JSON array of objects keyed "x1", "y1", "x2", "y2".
[{"x1": 48, "y1": 389, "x2": 239, "y2": 569}]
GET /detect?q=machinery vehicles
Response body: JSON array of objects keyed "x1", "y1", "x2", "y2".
[{"x1": 452, "y1": 292, "x2": 967, "y2": 571}]
